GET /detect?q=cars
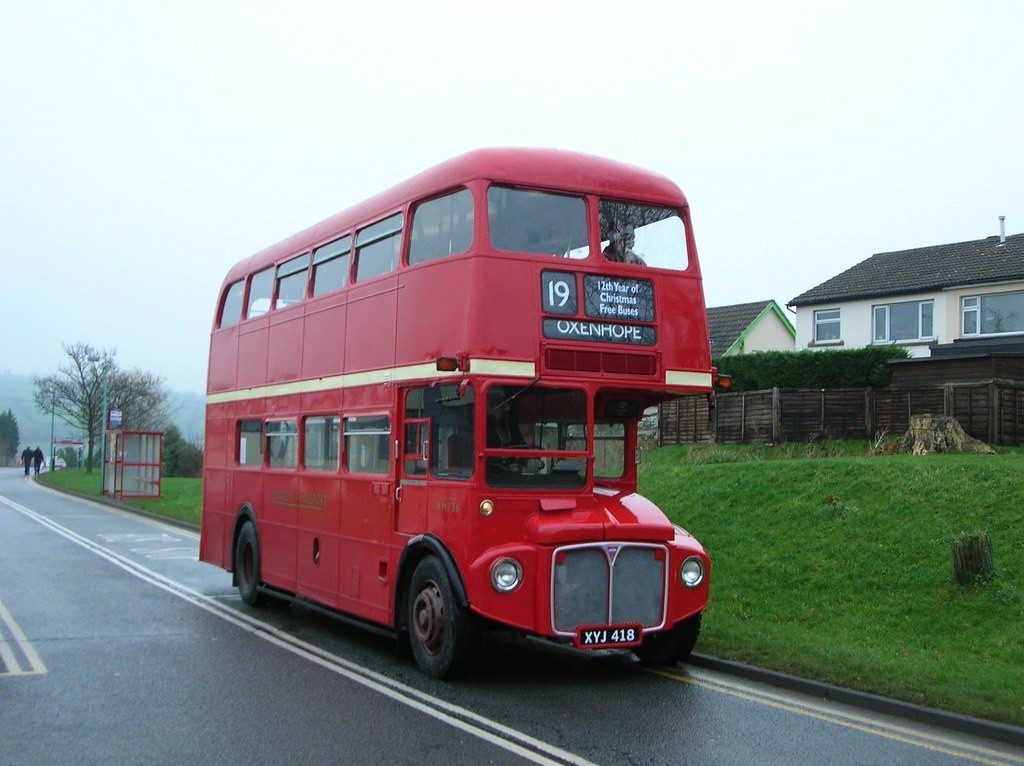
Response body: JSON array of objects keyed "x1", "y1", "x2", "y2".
[{"x1": 55, "y1": 459, "x2": 67, "y2": 469}]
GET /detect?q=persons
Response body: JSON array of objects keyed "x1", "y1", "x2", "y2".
[
  {"x1": 21, "y1": 445, "x2": 43, "y2": 475},
  {"x1": 603, "y1": 218, "x2": 646, "y2": 265}
]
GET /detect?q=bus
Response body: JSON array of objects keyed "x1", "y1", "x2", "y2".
[{"x1": 198, "y1": 146, "x2": 716, "y2": 685}]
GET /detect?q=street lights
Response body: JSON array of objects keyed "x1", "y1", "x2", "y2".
[
  {"x1": 89, "y1": 356, "x2": 108, "y2": 494},
  {"x1": 44, "y1": 388, "x2": 54, "y2": 471}
]
